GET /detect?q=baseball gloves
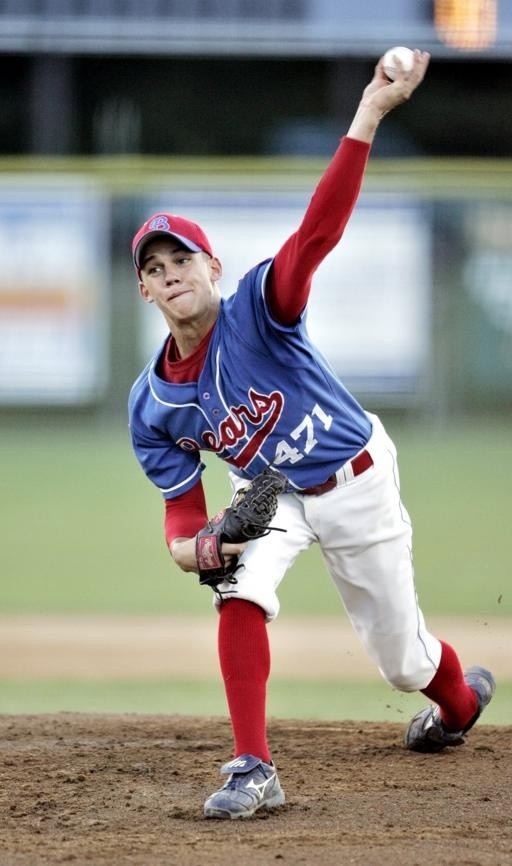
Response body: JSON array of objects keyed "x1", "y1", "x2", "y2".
[{"x1": 194, "y1": 466, "x2": 290, "y2": 598}]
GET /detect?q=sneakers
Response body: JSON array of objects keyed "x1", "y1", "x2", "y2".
[
  {"x1": 404, "y1": 667, "x2": 497, "y2": 753},
  {"x1": 204, "y1": 753, "x2": 285, "y2": 820}
]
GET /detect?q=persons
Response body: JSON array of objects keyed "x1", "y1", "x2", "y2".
[{"x1": 128, "y1": 47, "x2": 496, "y2": 820}]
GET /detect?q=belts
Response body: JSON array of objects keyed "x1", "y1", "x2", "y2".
[{"x1": 300, "y1": 450, "x2": 373, "y2": 494}]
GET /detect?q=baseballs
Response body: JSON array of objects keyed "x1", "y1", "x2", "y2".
[{"x1": 384, "y1": 47, "x2": 415, "y2": 81}]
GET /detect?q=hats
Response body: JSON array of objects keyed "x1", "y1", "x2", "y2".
[{"x1": 131, "y1": 212, "x2": 212, "y2": 282}]
